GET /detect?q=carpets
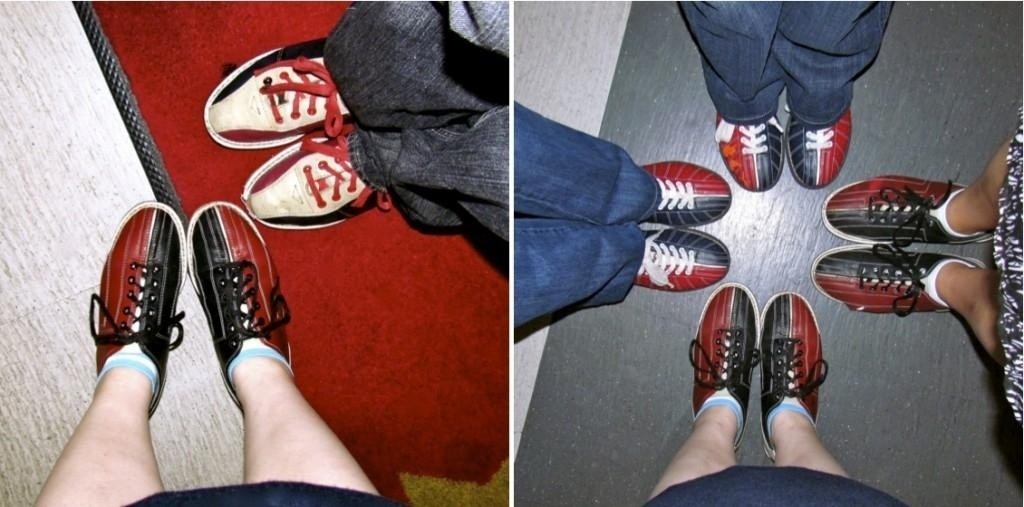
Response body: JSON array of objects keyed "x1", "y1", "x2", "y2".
[{"x1": 73, "y1": 0, "x2": 509, "y2": 507}]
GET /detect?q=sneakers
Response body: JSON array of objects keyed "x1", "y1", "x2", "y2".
[
  {"x1": 632, "y1": 226, "x2": 731, "y2": 294},
  {"x1": 809, "y1": 243, "x2": 989, "y2": 316},
  {"x1": 94, "y1": 199, "x2": 187, "y2": 424},
  {"x1": 239, "y1": 121, "x2": 389, "y2": 231},
  {"x1": 200, "y1": 37, "x2": 355, "y2": 150},
  {"x1": 757, "y1": 290, "x2": 823, "y2": 461},
  {"x1": 783, "y1": 103, "x2": 852, "y2": 190},
  {"x1": 184, "y1": 200, "x2": 285, "y2": 417},
  {"x1": 637, "y1": 159, "x2": 734, "y2": 228},
  {"x1": 712, "y1": 108, "x2": 785, "y2": 194},
  {"x1": 692, "y1": 281, "x2": 759, "y2": 454},
  {"x1": 819, "y1": 174, "x2": 994, "y2": 247}
]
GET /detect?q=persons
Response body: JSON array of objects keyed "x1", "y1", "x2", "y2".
[
  {"x1": 202, "y1": 2, "x2": 508, "y2": 242},
  {"x1": 677, "y1": 0, "x2": 901, "y2": 192},
  {"x1": 810, "y1": 114, "x2": 1022, "y2": 431},
  {"x1": 646, "y1": 280, "x2": 904, "y2": 506},
  {"x1": 514, "y1": 99, "x2": 733, "y2": 341},
  {"x1": 32, "y1": 199, "x2": 406, "y2": 507}
]
